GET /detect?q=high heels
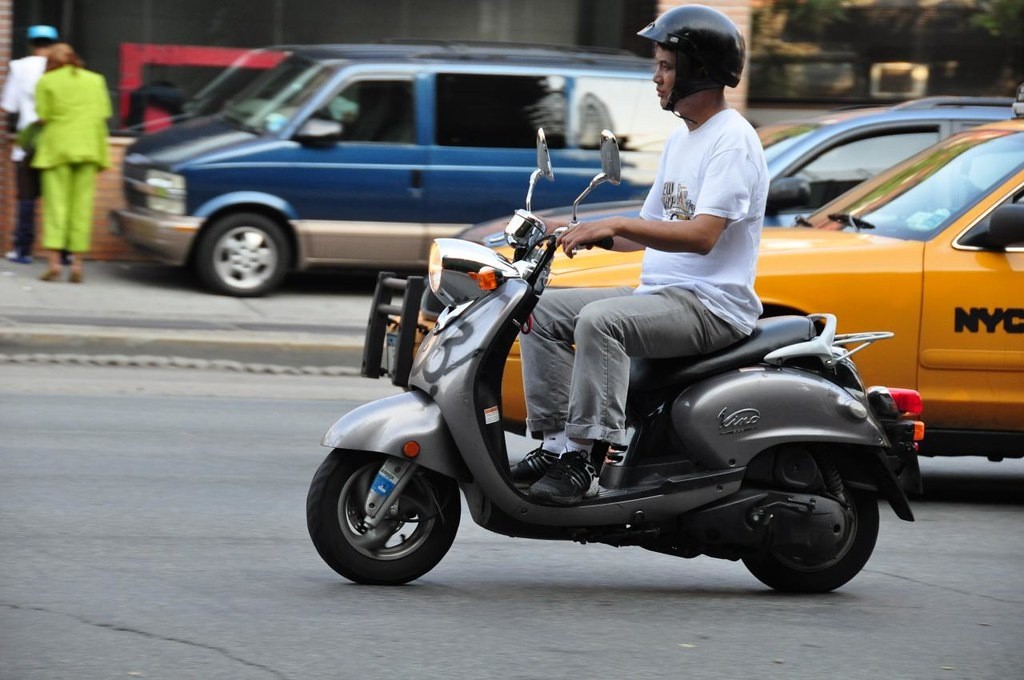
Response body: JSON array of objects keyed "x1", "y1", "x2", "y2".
[
  {"x1": 73, "y1": 266, "x2": 83, "y2": 282},
  {"x1": 40, "y1": 265, "x2": 62, "y2": 281}
]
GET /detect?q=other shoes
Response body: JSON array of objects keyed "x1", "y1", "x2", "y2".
[{"x1": 7, "y1": 250, "x2": 31, "y2": 263}]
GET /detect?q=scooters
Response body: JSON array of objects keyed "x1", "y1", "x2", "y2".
[{"x1": 306, "y1": 126, "x2": 925, "y2": 595}]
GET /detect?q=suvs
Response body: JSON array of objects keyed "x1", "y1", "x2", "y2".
[{"x1": 419, "y1": 95, "x2": 1024, "y2": 260}]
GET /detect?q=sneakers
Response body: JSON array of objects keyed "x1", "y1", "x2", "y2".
[
  {"x1": 530, "y1": 445, "x2": 600, "y2": 506},
  {"x1": 507, "y1": 443, "x2": 561, "y2": 489}
]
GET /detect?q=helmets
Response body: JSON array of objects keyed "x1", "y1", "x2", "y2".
[
  {"x1": 637, "y1": 3, "x2": 745, "y2": 87},
  {"x1": 25, "y1": 25, "x2": 58, "y2": 40}
]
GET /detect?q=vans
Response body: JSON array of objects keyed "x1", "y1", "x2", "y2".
[{"x1": 107, "y1": 43, "x2": 684, "y2": 299}]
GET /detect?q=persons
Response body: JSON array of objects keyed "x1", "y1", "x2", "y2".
[
  {"x1": 510, "y1": 4, "x2": 770, "y2": 506},
  {"x1": 0, "y1": 25, "x2": 186, "y2": 284}
]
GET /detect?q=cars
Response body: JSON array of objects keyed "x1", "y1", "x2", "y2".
[{"x1": 361, "y1": 121, "x2": 1024, "y2": 463}]
